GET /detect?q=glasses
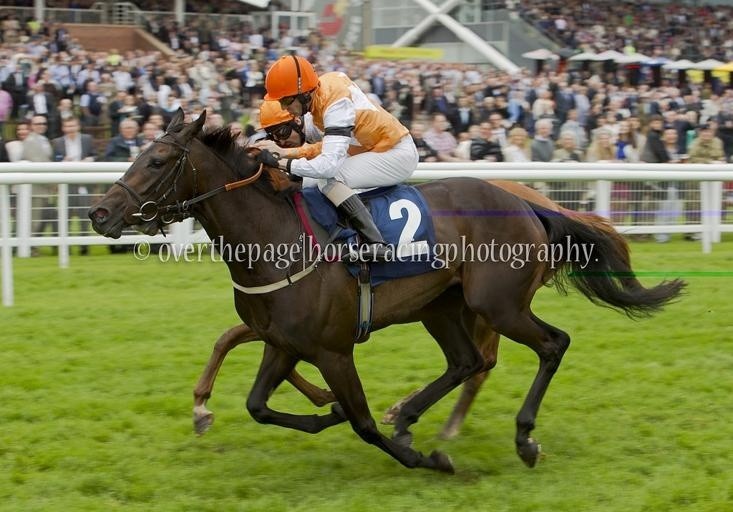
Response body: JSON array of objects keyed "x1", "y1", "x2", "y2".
[
  {"x1": 267, "y1": 127, "x2": 291, "y2": 140},
  {"x1": 281, "y1": 94, "x2": 298, "y2": 105}
]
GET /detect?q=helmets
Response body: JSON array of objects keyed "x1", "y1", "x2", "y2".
[
  {"x1": 255, "y1": 94, "x2": 294, "y2": 130},
  {"x1": 263, "y1": 55, "x2": 319, "y2": 101}
]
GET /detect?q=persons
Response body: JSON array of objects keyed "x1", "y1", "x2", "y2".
[{"x1": 0, "y1": 4, "x2": 731, "y2": 260}]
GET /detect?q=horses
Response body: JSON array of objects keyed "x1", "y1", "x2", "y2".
[{"x1": 86, "y1": 106, "x2": 690, "y2": 476}]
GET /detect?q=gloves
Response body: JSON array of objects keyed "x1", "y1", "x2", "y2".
[{"x1": 256, "y1": 150, "x2": 292, "y2": 174}]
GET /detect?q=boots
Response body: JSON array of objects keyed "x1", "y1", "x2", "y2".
[{"x1": 338, "y1": 194, "x2": 392, "y2": 260}]
